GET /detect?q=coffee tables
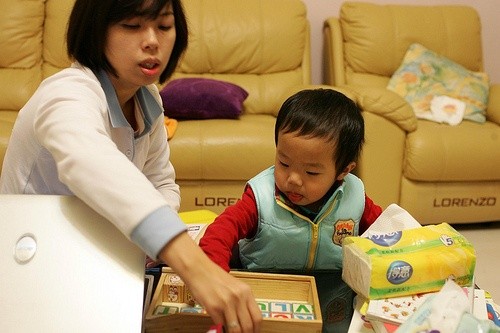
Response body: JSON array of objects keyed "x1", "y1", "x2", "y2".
[{"x1": 145, "y1": 263, "x2": 481, "y2": 333}]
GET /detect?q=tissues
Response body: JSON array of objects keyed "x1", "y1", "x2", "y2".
[
  {"x1": 342, "y1": 203, "x2": 477, "y2": 299},
  {"x1": 394, "y1": 279, "x2": 493, "y2": 333}
]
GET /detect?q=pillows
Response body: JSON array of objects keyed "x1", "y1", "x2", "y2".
[
  {"x1": 386, "y1": 42, "x2": 491, "y2": 124},
  {"x1": 159, "y1": 78, "x2": 248, "y2": 121}
]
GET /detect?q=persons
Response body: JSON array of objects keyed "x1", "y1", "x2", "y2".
[
  {"x1": 197, "y1": 86, "x2": 383, "y2": 276},
  {"x1": 0, "y1": 0, "x2": 263, "y2": 333}
]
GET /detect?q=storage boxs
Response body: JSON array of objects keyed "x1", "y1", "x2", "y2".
[{"x1": 143, "y1": 266, "x2": 323, "y2": 333}]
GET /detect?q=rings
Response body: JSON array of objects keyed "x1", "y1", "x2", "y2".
[{"x1": 228, "y1": 320, "x2": 238, "y2": 328}]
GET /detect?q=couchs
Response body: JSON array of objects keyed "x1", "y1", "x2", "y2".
[
  {"x1": 322, "y1": 1, "x2": 500, "y2": 225},
  {"x1": 0, "y1": 0, "x2": 355, "y2": 217}
]
